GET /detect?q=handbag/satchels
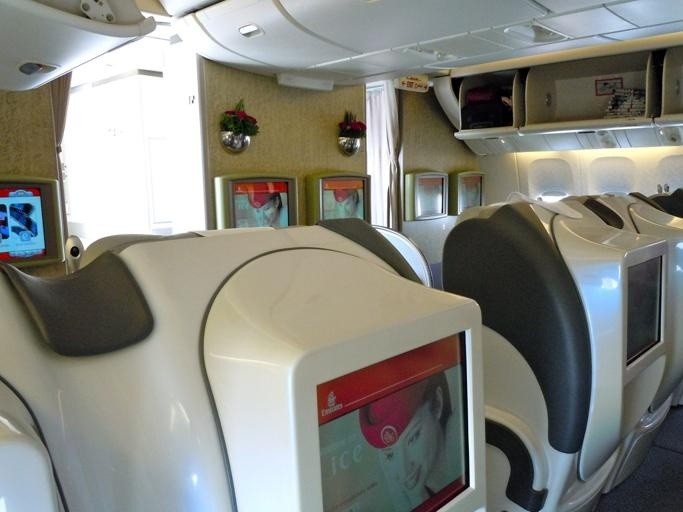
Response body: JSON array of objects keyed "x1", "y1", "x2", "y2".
[{"x1": 461, "y1": 84, "x2": 513, "y2": 129}]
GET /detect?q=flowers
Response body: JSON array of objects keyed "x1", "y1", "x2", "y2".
[
  {"x1": 217, "y1": 94, "x2": 259, "y2": 137},
  {"x1": 338, "y1": 107, "x2": 367, "y2": 140}
]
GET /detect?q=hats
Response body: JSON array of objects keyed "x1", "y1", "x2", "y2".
[
  {"x1": 333, "y1": 190, "x2": 355, "y2": 202},
  {"x1": 247, "y1": 193, "x2": 275, "y2": 207},
  {"x1": 359, "y1": 379, "x2": 430, "y2": 450}
]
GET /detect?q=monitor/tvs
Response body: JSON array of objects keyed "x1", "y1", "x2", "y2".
[
  {"x1": 306, "y1": 172, "x2": 371, "y2": 225},
  {"x1": 0, "y1": 175, "x2": 66, "y2": 268},
  {"x1": 627, "y1": 255, "x2": 661, "y2": 362},
  {"x1": 405, "y1": 172, "x2": 448, "y2": 221},
  {"x1": 450, "y1": 170, "x2": 488, "y2": 216},
  {"x1": 316, "y1": 328, "x2": 465, "y2": 512},
  {"x1": 214, "y1": 171, "x2": 299, "y2": 230}
]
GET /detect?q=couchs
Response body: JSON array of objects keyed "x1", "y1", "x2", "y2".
[
  {"x1": 2, "y1": 216, "x2": 487, "y2": 512},
  {"x1": 442, "y1": 190, "x2": 683, "y2": 511}
]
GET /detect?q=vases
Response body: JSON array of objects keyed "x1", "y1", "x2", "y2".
[
  {"x1": 339, "y1": 135, "x2": 360, "y2": 157},
  {"x1": 220, "y1": 128, "x2": 250, "y2": 153}
]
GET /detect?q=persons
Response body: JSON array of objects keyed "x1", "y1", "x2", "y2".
[
  {"x1": 355, "y1": 371, "x2": 464, "y2": 506},
  {"x1": 251, "y1": 189, "x2": 288, "y2": 230},
  {"x1": 332, "y1": 187, "x2": 362, "y2": 218}
]
